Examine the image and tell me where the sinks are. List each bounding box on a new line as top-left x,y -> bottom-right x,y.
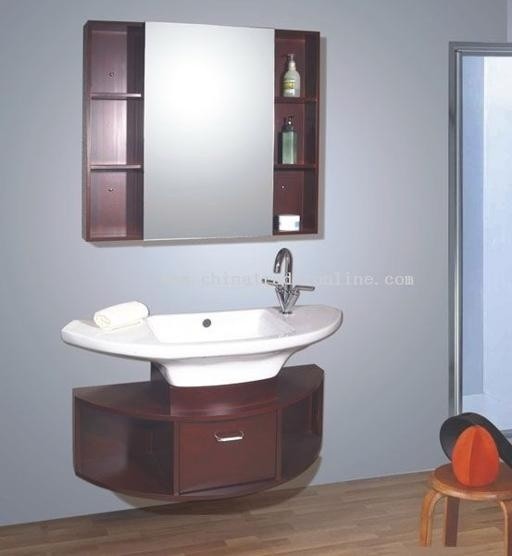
61,304 -> 344,388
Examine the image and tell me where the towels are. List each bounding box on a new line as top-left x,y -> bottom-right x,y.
94,300 -> 149,332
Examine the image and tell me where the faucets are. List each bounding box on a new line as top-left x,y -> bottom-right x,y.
261,248 -> 315,313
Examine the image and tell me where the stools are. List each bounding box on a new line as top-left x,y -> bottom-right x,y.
418,460 -> 511,556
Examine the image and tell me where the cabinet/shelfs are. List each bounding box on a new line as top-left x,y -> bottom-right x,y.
71,364 -> 325,502
273,28 -> 320,236
82,20 -> 144,243
